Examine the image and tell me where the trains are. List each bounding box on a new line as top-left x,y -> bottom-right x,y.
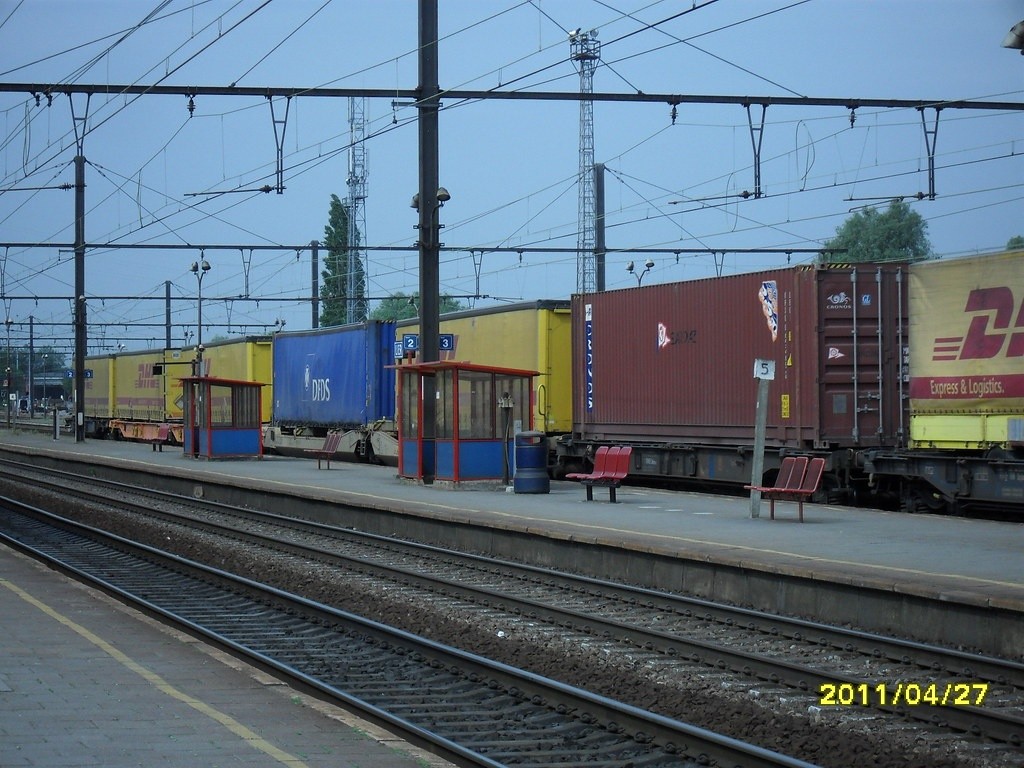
70,234 -> 1023,522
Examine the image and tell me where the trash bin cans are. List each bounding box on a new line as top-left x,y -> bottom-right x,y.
514,431 -> 550,494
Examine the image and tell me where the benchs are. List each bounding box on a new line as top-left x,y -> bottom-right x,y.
302,433 -> 342,469
565,446 -> 632,504
743,457 -> 825,523
150,422 -> 171,452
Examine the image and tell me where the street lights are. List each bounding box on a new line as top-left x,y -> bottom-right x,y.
4,317 -> 15,428
410,187 -> 451,364
190,260 -> 212,377
